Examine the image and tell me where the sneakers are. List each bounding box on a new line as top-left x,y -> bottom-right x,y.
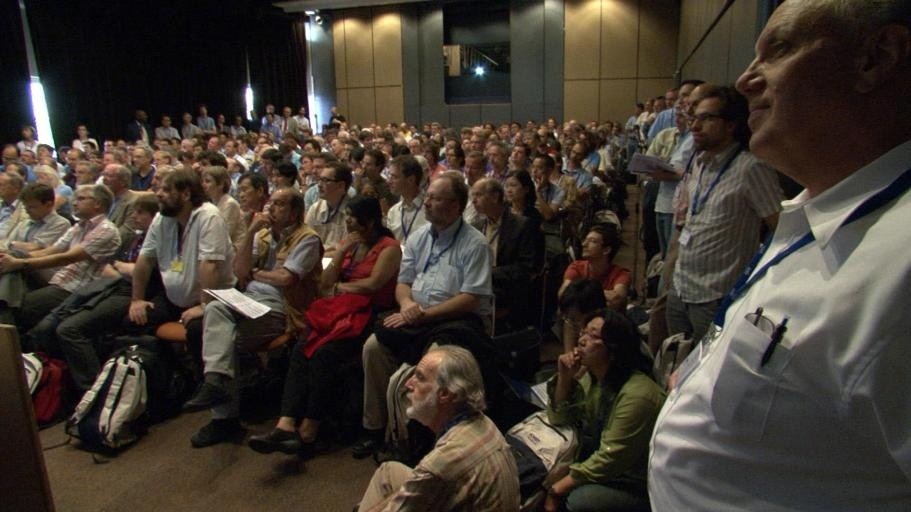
183,381 -> 232,412
191,417 -> 244,447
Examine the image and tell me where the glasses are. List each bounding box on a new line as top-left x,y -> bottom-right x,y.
316,176 -> 336,182
78,196 -> 95,201
579,328 -> 602,339
745,306 -> 774,337
688,111 -> 722,121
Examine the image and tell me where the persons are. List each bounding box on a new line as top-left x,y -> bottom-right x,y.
351,170 -> 494,463
562,142 -> 593,197
556,227 -> 631,352
228,104 -> 321,187
0,185 -> 122,331
237,172 -> 271,238
385,156 -> 429,244
627,79 -> 678,257
305,163 -> 353,259
0,182 -> 70,317
461,150 -> 488,232
0,172 -> 29,247
472,177 -> 535,305
530,153 -> 566,279
201,166 -> 247,255
646,1 -> 911,511
124,168 -> 238,382
545,152 -> 577,212
248,193 -> 402,455
30,194 -> 160,383
100,163 -> 140,227
304,152 -> 355,217
33,164 -> 73,223
323,106 -> 622,156
546,306 -> 667,512
502,168 -> 541,237
0,110 -> 228,165
666,85 -> 787,349
357,344 -> 521,511
73,159 -> 100,187
182,187 -> 325,446
559,280 -> 655,377
351,151 -> 392,207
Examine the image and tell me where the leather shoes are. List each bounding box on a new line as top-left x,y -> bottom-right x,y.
352,437 -> 371,454
247,427 -> 297,453
280,439 -> 316,457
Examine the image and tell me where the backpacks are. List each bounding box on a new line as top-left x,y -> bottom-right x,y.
46,343 -> 157,465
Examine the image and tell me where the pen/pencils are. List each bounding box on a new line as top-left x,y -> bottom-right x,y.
758,317 -> 789,367
